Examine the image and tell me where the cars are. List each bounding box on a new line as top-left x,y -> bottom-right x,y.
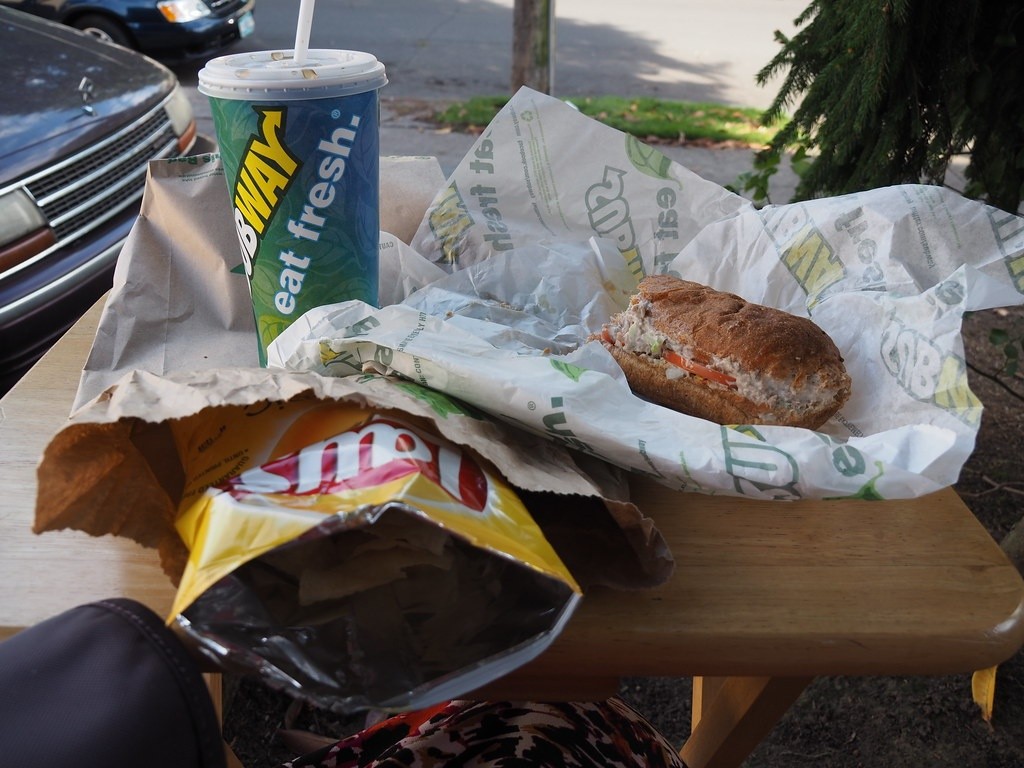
0,4 -> 219,399
6,0 -> 262,68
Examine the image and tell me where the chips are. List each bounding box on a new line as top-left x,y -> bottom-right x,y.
260,521 -> 458,607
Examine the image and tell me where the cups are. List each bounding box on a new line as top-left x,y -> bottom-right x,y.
198,49 -> 389,370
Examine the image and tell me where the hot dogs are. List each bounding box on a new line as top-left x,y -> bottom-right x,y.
596,281 -> 851,428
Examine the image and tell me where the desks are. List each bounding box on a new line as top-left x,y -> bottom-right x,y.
0,287 -> 1024,768
